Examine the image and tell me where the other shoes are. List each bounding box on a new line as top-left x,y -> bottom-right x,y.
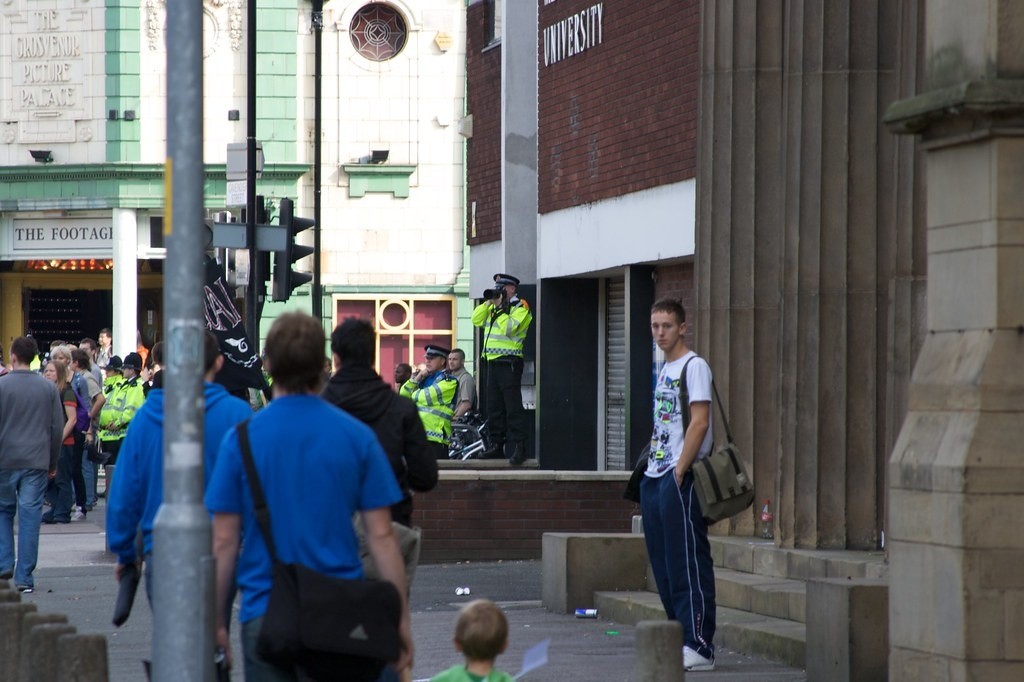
41,508 -> 55,524
682,646 -> 715,671
52,510 -> 72,524
510,447 -> 528,464
478,442 -> 505,459
0,570 -> 13,580
70,511 -> 87,522
17,585 -> 33,593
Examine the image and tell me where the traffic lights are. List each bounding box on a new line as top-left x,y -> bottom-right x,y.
272,196 -> 314,304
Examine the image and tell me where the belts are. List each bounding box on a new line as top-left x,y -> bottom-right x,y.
484,360 -> 523,368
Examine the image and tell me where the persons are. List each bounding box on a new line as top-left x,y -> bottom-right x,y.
398,599 -> 514,682
394,344 -> 475,459
471,273 -> 533,465
0,337 -> 64,591
105,310 -> 439,682
0,328 -> 167,524
639,298 -> 715,671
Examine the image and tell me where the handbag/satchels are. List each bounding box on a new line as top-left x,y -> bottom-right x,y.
255,562 -> 402,682
691,444 -> 756,527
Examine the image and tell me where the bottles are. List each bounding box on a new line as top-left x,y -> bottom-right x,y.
761,499 -> 773,538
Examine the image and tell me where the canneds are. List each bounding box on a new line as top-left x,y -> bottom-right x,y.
575,608 -> 598,618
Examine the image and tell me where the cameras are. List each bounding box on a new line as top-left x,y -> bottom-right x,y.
484,286 -> 507,301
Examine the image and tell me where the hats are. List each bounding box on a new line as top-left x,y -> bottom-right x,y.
100,356 -> 123,370
424,345 -> 451,360
494,274 -> 520,287
86,445 -> 112,465
121,352 -> 142,371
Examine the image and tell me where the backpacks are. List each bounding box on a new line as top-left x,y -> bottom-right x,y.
60,386 -> 90,432
